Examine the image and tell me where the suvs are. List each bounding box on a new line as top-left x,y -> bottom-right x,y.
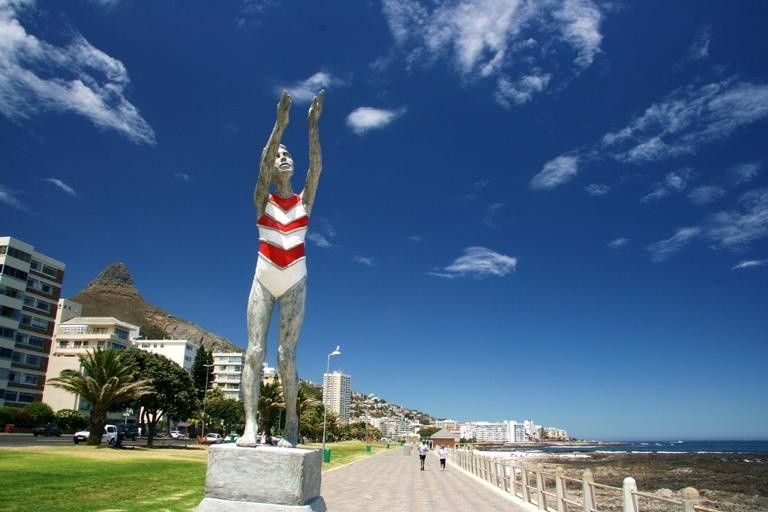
74,424 -> 118,445
117,425 -> 138,441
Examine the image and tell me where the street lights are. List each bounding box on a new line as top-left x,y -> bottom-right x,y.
204,365 -> 213,417
322,345 -> 342,459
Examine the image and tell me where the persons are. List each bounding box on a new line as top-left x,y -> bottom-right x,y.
235,88 -> 327,447
417,439 -> 429,470
437,444 -> 448,471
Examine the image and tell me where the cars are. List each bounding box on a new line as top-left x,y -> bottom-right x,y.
172,431 -> 186,439
224,433 -> 240,442
33,423 -> 62,437
202,433 -> 222,444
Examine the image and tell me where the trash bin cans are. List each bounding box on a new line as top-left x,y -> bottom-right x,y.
324,448 -> 330,463
367,445 -> 372,452
387,444 -> 389,448
